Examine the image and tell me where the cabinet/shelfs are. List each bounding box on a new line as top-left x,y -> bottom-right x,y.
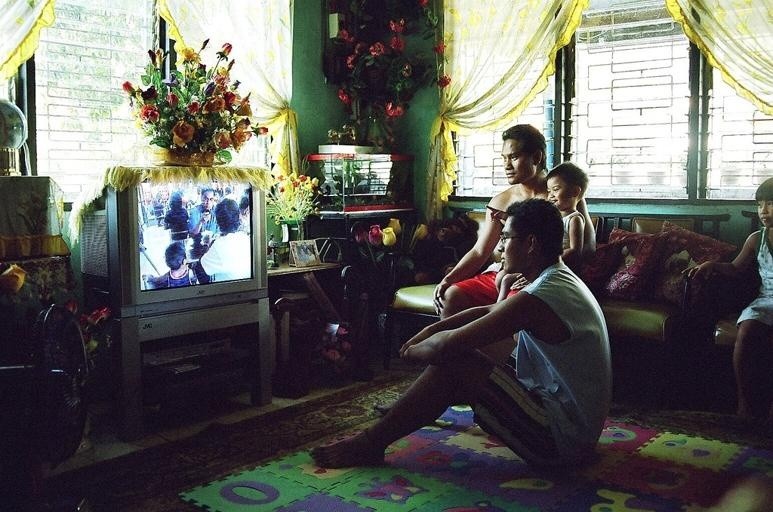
307,145 -> 413,243
114,297 -> 274,443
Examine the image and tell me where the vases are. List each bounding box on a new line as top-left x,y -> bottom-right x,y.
376,311 -> 393,335
287,226 -> 304,241
164,150 -> 215,167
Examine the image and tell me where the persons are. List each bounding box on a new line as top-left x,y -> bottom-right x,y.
680,177 -> 773,419
308,197 -> 614,470
433,122 -> 597,321
142,184 -> 251,288
485,161 -> 590,303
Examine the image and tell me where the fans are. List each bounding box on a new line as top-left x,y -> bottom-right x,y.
26,305 -> 89,512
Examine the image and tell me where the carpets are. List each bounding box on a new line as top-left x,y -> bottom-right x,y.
0,366 -> 773,512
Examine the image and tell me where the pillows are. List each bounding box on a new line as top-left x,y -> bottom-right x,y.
648,220 -> 736,308
565,243 -> 618,292
603,226 -> 663,300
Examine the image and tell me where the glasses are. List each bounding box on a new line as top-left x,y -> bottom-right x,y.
500,235 -> 521,243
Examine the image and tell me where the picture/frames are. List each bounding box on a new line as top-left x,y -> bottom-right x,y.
289,239 -> 322,268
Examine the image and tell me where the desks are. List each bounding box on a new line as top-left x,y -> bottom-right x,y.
268,261 -> 342,383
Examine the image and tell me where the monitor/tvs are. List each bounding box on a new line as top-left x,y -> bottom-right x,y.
80,166 -> 268,318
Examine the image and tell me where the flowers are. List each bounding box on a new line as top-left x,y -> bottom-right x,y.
317,319 -> 352,387
350,218 -> 450,287
325,0 -> 449,153
267,171 -> 322,225
123,37 -> 268,163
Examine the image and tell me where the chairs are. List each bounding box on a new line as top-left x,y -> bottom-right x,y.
597,213 -> 732,384
710,212 -> 758,402
388,211 -> 601,383
